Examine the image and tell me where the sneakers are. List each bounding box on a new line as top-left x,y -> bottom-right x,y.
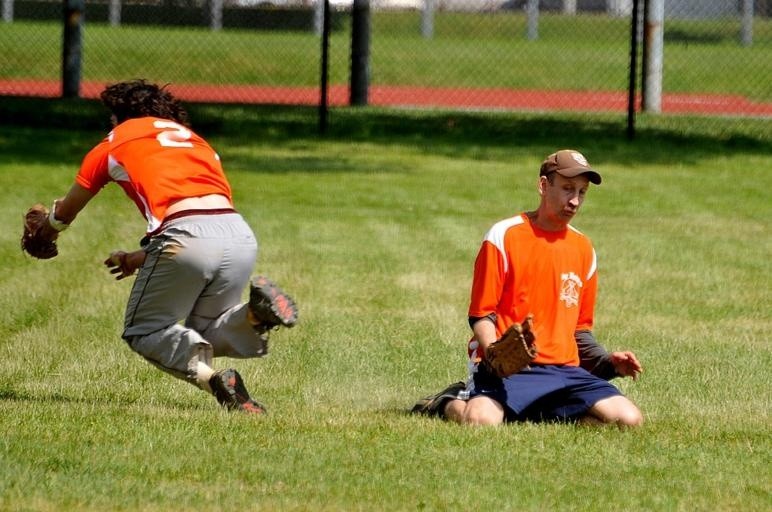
209,368 -> 267,414
249,275 -> 299,328
410,381 -> 467,417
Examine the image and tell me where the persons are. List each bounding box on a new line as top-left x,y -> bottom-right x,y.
408,149 -> 644,430
22,78 -> 299,414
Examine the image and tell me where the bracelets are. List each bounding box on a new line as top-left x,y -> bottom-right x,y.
47,199 -> 70,231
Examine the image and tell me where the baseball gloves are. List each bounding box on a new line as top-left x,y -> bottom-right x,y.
488,313 -> 536,379
21,203 -> 58,259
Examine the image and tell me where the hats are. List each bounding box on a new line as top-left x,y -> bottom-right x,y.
540,150 -> 601,186
